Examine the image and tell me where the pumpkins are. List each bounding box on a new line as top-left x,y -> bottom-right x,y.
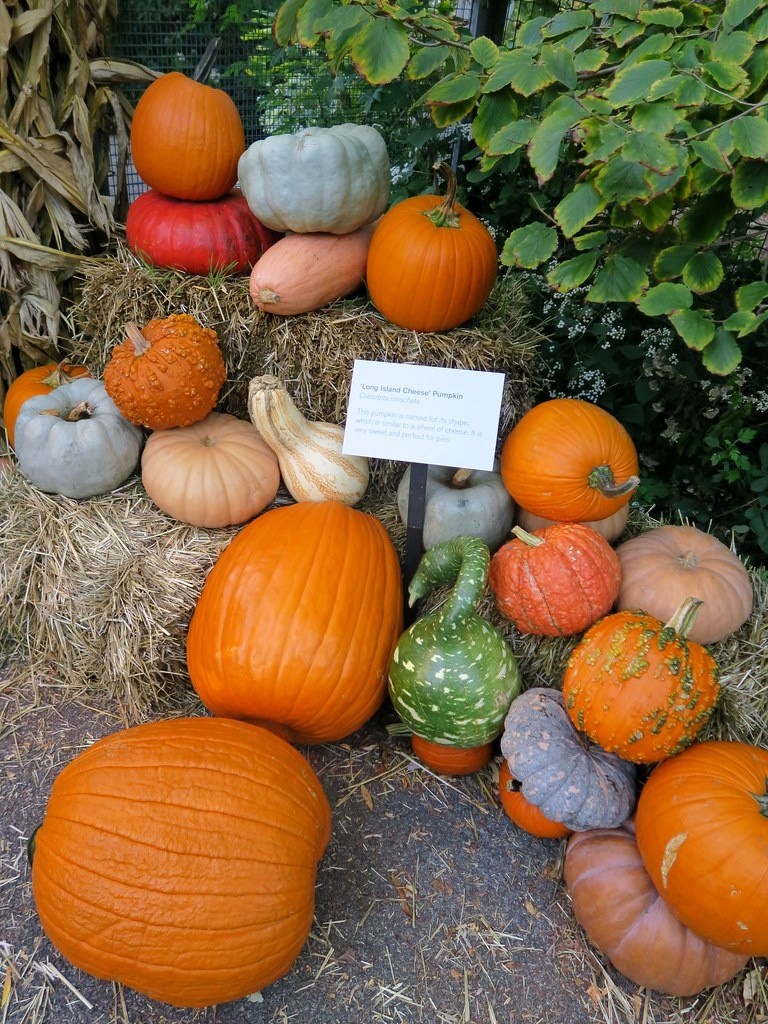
4,35 -> 768,1009
